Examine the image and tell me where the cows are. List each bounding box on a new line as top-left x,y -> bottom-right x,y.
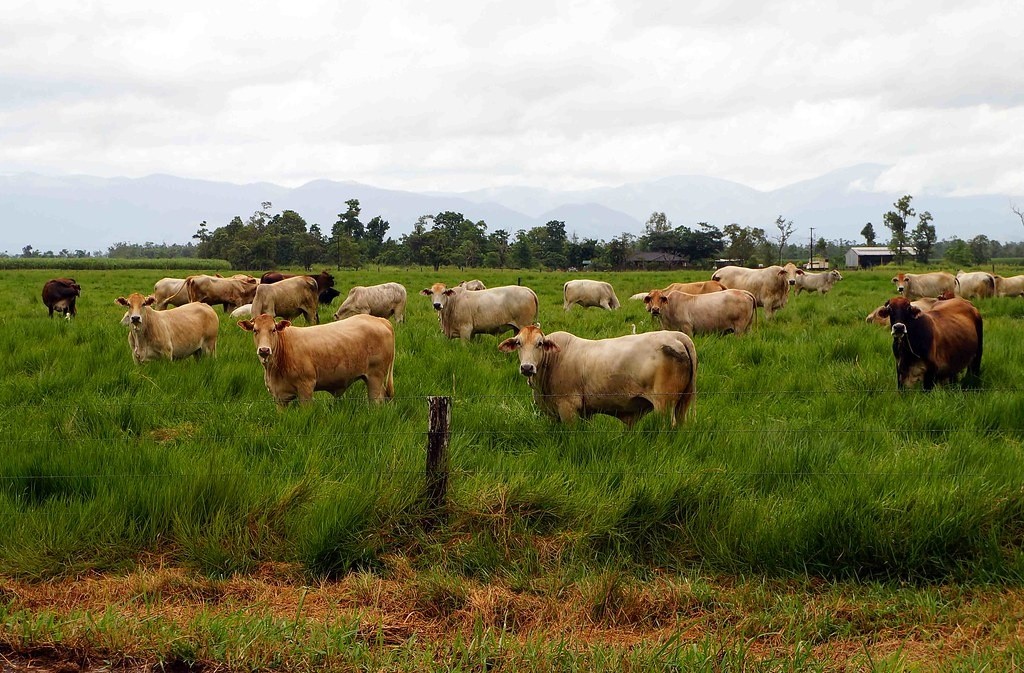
419,283 -> 540,344
497,322 -> 698,432
456,279 -> 486,291
152,270 -> 340,314
956,269 -> 996,301
878,296 -> 985,394
42,278 -> 81,320
113,293 -> 219,366
563,279 -> 621,314
795,269 -> 843,296
333,283 -> 406,324
712,262 -> 806,321
991,274 -> 1024,298
643,281 -> 760,339
237,314 -> 396,413
863,297 -> 939,327
891,271 -> 961,301
